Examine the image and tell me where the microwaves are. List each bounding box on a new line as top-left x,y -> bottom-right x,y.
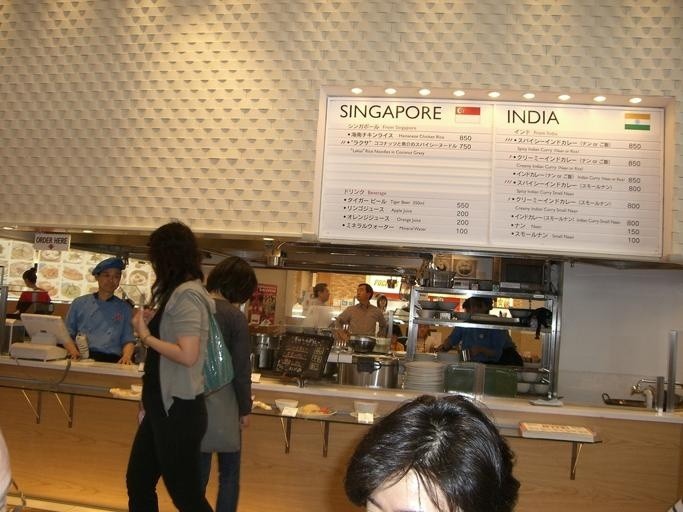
501,259 -> 551,291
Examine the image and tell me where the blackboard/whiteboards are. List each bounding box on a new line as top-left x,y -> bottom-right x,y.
273,332 -> 334,381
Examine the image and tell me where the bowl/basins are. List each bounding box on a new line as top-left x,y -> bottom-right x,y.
439,302 -> 458,310
346,339 -> 376,354
534,384 -> 550,395
516,383 -> 531,393
416,310 -> 435,319
523,373 -> 537,381
516,372 -> 523,382
275,399 -> 298,410
420,300 -> 442,309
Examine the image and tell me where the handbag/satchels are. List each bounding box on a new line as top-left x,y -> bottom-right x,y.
202,315 -> 234,396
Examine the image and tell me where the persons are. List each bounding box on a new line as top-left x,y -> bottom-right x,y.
125,297 -> 157,327
127,222 -> 213,512
199,256 -> 258,509
302,283 -> 330,316
14,268 -> 51,342
377,294 -> 387,313
344,393 -> 519,511
63,257 -> 137,366
335,283 -> 388,341
405,323 -> 435,353
431,296 -> 523,365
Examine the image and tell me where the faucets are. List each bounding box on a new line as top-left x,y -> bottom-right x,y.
630,384 -> 653,408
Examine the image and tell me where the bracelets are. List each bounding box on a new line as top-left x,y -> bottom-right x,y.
141,335 -> 150,349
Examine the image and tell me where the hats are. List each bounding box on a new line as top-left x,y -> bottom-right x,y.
92,257 -> 125,275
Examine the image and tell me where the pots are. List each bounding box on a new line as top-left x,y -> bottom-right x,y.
252,335 -> 276,369
337,355 -> 400,389
429,268 -> 456,288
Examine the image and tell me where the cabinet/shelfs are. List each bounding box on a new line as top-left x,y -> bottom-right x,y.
406,286 -> 557,397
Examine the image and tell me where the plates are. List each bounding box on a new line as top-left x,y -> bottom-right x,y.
403,361 -> 445,392
304,410 -> 339,418
349,411 -> 380,419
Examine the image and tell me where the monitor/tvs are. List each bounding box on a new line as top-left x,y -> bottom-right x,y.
20,313 -> 74,347
